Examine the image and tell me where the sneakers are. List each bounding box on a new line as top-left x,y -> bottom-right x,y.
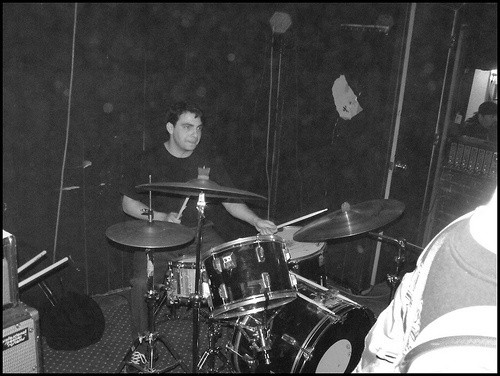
126,343 -> 157,374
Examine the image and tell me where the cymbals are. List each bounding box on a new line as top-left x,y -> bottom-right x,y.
293,198 -> 406,241
106,220 -> 194,248
137,177 -> 268,201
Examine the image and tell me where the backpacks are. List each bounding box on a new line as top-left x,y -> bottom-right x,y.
20,270 -> 104,351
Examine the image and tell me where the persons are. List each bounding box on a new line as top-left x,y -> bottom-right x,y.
351,188 -> 497,373
122,102 -> 278,373
321,71 -> 413,283
447,100 -> 497,163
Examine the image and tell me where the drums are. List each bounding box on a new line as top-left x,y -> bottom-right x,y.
257,226 -> 327,288
200,235 -> 298,320
229,282 -> 376,373
168,256 -> 212,308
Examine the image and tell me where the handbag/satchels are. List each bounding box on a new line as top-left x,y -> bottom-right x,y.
352,267 -> 408,373
